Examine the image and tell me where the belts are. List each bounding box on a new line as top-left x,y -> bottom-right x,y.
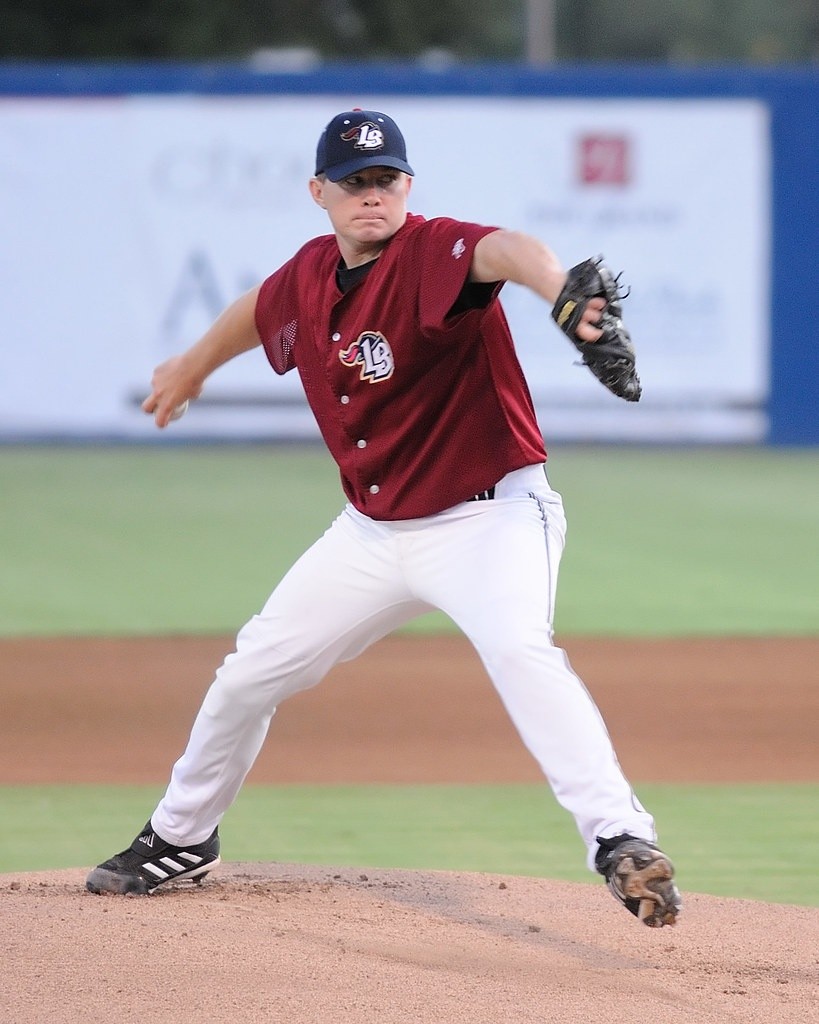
467,486 -> 494,501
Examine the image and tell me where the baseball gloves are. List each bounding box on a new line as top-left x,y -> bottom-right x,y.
549,255 -> 643,403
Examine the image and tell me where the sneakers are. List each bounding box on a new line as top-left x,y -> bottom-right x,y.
88,821 -> 221,894
594,835 -> 682,928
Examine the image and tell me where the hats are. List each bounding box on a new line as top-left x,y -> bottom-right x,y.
316,109 -> 415,182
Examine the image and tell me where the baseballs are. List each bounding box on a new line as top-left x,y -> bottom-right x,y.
153,398 -> 190,423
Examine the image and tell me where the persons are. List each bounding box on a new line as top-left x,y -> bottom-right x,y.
84,106 -> 680,929
